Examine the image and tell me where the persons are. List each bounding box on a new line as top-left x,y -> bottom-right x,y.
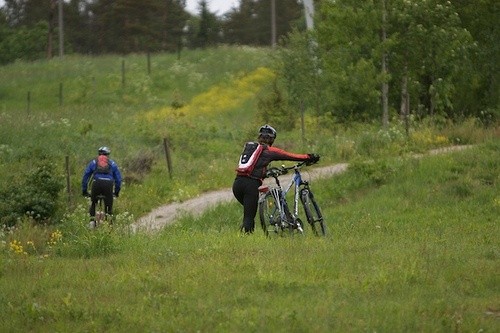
81,146 -> 122,231
232,125 -> 320,237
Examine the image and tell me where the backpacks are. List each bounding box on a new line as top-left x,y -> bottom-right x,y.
96,155 -> 114,175
238,141 -> 268,178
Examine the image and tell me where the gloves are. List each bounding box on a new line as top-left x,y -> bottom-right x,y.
114,191 -> 119,197
306,153 -> 320,163
83,192 -> 90,197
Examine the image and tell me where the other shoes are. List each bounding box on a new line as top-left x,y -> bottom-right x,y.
90,221 -> 95,229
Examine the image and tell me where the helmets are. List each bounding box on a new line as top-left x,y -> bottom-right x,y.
98,146 -> 111,154
258,124 -> 276,138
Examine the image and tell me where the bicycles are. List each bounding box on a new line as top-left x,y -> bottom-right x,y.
258,155 -> 324,239
84,194 -> 116,227
